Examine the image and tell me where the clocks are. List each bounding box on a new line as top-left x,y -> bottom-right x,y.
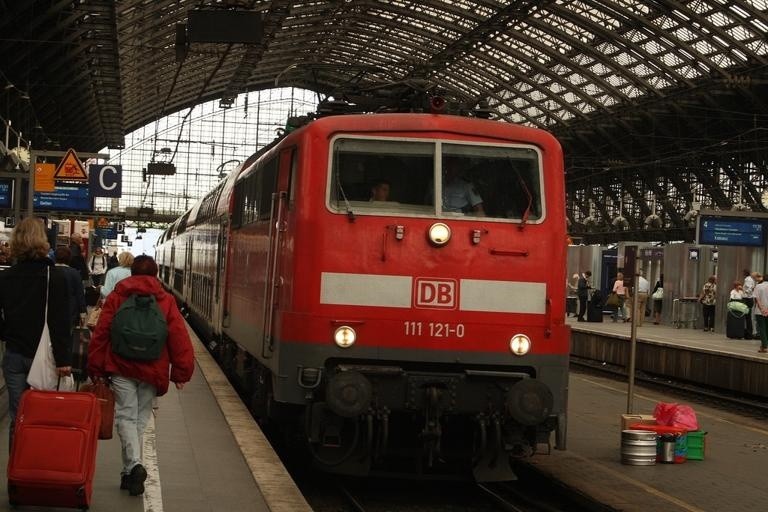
10,147 -> 30,169
759,189 -> 768,212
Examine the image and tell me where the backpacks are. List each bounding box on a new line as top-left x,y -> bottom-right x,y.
108,293 -> 169,362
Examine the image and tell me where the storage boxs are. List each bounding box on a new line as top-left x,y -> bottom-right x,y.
658,427 -> 708,461
622,414 -> 656,430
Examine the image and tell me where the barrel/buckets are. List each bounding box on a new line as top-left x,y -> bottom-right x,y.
622,430 -> 657,465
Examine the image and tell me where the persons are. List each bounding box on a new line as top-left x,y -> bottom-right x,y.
756,274 -> 767,352
89,252 -> 196,495
730,266 -> 766,340
0,218 -> 74,460
697,276 -> 717,332
53,227 -> 136,351
366,179 -> 393,202
424,161 -> 484,220
566,266 -> 666,327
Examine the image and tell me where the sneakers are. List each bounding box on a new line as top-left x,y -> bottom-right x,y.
577,316 -> 715,332
120,464 -> 148,496
758,347 -> 768,353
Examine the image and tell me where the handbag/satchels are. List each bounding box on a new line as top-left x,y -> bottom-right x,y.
78,376 -> 115,440
652,287 -> 664,300
85,305 -> 103,333
590,290 -> 603,306
727,301 -> 750,319
25,322 -> 76,393
605,292 -> 620,307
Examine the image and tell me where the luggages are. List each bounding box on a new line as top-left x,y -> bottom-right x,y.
587,300 -> 603,323
71,326 -> 93,384
6,368 -> 102,512
565,296 -> 578,313
726,310 -> 745,340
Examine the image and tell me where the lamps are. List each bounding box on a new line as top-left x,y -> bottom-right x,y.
567,174 -> 748,229
1,119 -> 31,173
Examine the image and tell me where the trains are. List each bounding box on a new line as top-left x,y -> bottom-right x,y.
152,85 -> 569,486
